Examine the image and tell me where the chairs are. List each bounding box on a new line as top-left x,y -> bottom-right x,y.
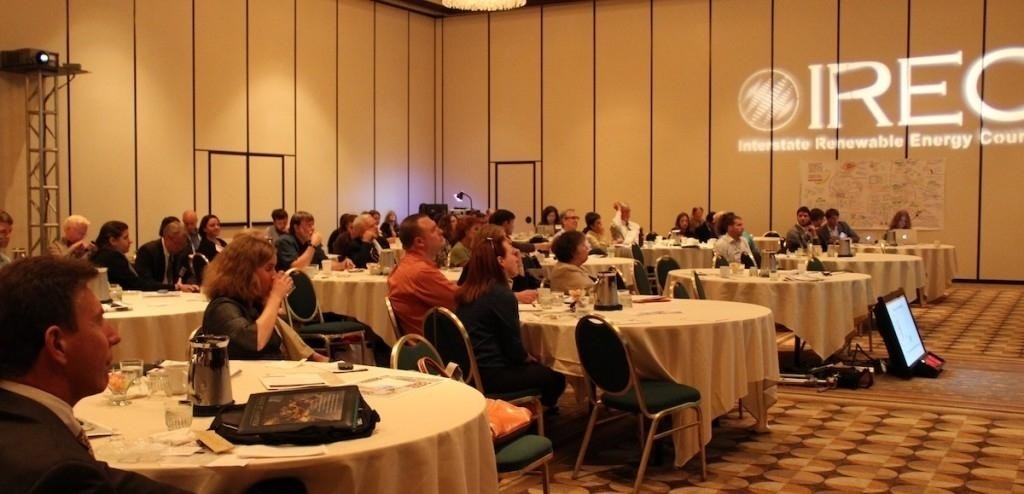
283,266 -> 368,366
193,252 -> 211,285
655,256 -> 682,294
390,332 -> 554,494
670,283 -> 690,299
572,315 -> 707,494
633,258 -> 649,294
424,305 -> 546,437
384,298 -> 404,339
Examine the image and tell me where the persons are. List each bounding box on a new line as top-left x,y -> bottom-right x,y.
551,231 -> 598,292
786,206 -> 821,253
159,216 -> 181,237
438,214 -> 465,246
609,200 -> 640,246
369,210 -> 380,225
553,209 -> 579,238
456,235 -> 567,414
196,215 -> 227,261
447,218 -> 487,267
45,215 -> 95,256
489,209 -> 553,253
182,210 -> 202,250
343,214 -> 391,268
201,233 -> 295,360
277,210 -> 356,274
385,214 -> 463,344
380,211 -> 400,238
688,208 -> 705,234
671,212 -> 693,238
0,210 -> 14,262
694,212 -> 719,243
884,210 -> 911,240
264,208 -> 289,238
0,253 -> 166,493
93,221 -> 138,290
333,215 -> 358,254
536,206 -> 560,236
712,216 -> 758,268
810,208 -> 829,245
582,212 -> 609,251
135,222 -> 200,291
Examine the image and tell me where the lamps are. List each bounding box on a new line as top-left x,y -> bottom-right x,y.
452,191 -> 479,214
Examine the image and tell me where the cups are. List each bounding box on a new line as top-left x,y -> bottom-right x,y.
119,359 -> 144,385
322,259 -> 331,274
148,371 -> 170,401
750,267 -> 757,277
276,270 -> 295,297
165,400 -> 193,433
620,293 -> 631,308
720,265 -> 730,278
163,363 -> 189,395
104,368 -> 136,405
536,287 -> 551,305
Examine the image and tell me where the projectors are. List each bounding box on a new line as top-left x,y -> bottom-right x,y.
0,47 -> 60,74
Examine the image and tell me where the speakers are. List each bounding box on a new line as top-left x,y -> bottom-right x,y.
418,203 -> 448,221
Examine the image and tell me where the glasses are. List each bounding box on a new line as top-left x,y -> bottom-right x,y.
486,236 -> 495,249
564,216 -> 579,220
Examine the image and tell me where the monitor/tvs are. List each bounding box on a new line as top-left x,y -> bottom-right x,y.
873,287 -> 927,380
